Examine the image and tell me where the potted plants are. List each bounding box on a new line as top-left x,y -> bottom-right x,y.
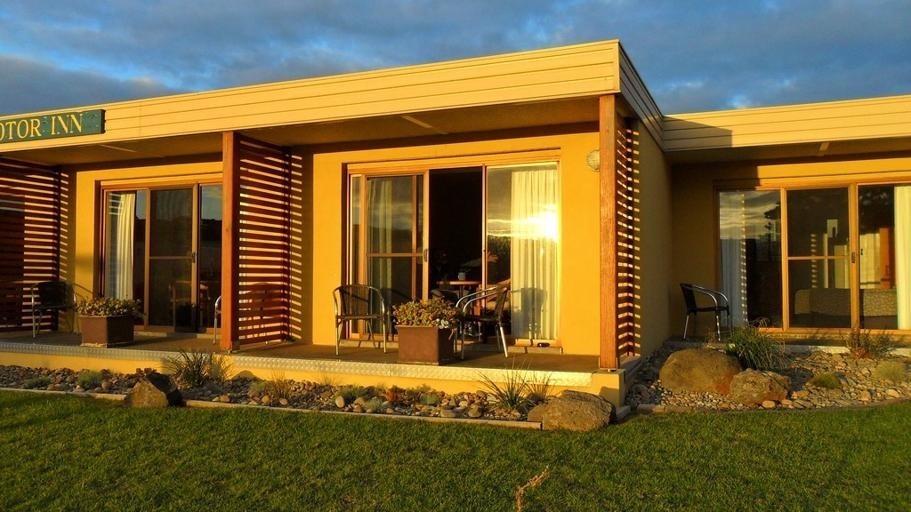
390,299 -> 461,365
77,296 -> 142,348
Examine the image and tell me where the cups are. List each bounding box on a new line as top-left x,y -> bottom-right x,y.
458,271 -> 465,280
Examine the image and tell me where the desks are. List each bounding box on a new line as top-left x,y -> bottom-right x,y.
436,281 -> 479,298
174,279 -> 220,330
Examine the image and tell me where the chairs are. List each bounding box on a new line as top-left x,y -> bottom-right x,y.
213,284 -> 270,344
679,283 -> 733,342
332,284 -> 386,356
453,283 -> 510,361
31,282 -> 77,339
